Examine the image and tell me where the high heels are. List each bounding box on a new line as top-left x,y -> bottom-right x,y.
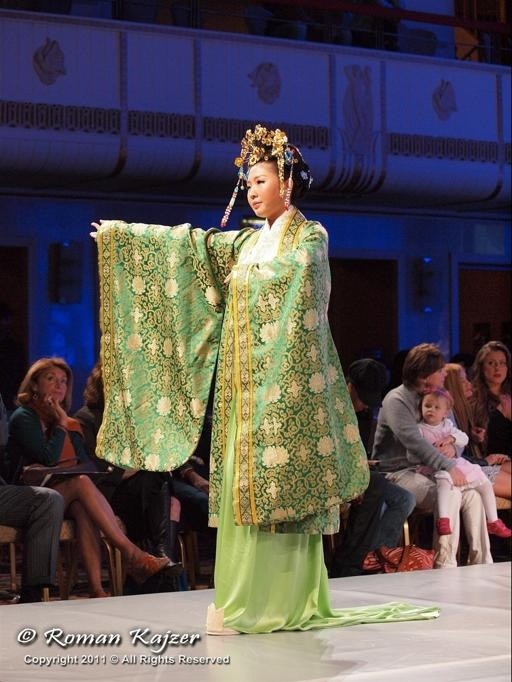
131,555 -> 169,584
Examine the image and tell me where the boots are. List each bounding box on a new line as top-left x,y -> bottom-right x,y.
153,482 -> 184,577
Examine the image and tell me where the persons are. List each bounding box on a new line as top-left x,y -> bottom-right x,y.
88,125 -> 331,634
343,341 -> 512,572
0,357 -> 209,603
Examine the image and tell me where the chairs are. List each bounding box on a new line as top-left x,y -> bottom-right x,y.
1,464 -> 512,601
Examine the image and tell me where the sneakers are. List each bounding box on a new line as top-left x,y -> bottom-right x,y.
487,519 -> 512,537
435,518 -> 451,535
207,602 -> 241,635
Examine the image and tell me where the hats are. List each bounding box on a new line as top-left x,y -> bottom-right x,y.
347,358 -> 391,408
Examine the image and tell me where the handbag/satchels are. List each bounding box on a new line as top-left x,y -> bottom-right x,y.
19,458 -> 106,487
